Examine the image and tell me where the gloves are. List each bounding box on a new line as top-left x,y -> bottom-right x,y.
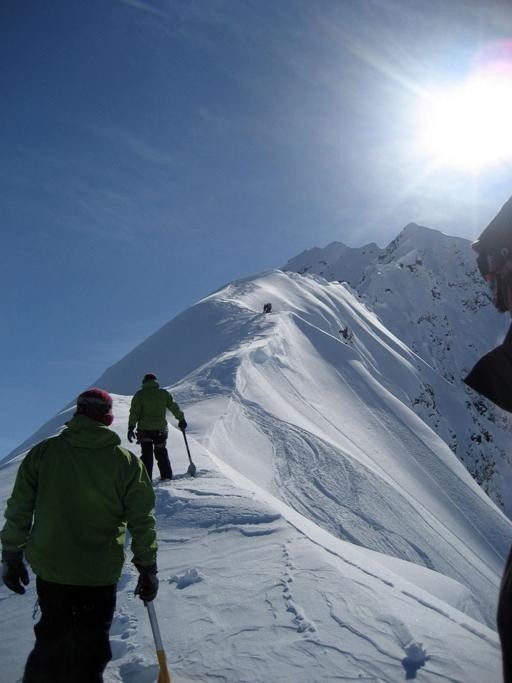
133,565 -> 160,603
177,420 -> 188,430
127,429 -> 136,443
0,550 -> 29,595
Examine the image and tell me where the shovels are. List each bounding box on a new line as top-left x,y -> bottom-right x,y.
183,429 -> 196,476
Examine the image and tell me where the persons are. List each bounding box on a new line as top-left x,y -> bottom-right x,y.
127,373 -> 188,482
0,387 -> 160,683
460,196 -> 512,683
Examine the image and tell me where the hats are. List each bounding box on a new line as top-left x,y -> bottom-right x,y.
74,388 -> 113,426
144,373 -> 157,380
